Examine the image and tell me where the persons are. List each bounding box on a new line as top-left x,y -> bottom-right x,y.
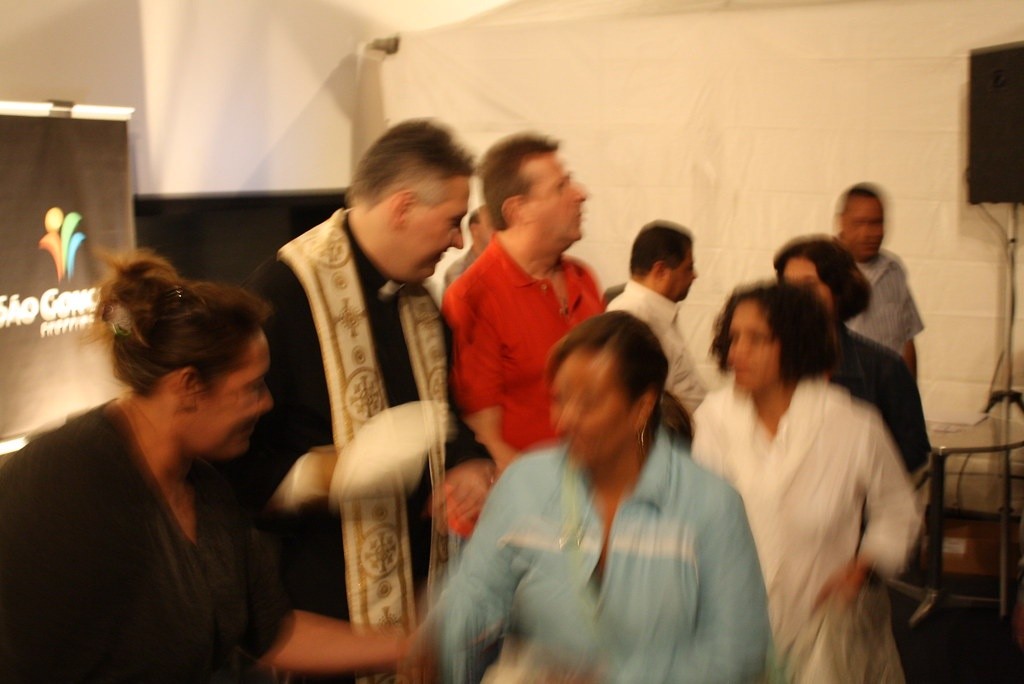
228,115 -> 495,683
440,133 -> 603,485
691,282 -> 922,684
607,221 -> 708,413
443,208 -> 493,283
400,311 -> 768,684
836,184 -> 923,384
772,236 -> 932,560
1,255 -> 407,684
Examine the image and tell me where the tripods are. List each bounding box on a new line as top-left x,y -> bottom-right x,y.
909,202 -> 1024,621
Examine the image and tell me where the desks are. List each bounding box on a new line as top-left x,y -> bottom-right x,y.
892,417 -> 1024,628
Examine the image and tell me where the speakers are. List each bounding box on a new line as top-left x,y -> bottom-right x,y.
966,42 -> 1024,205
